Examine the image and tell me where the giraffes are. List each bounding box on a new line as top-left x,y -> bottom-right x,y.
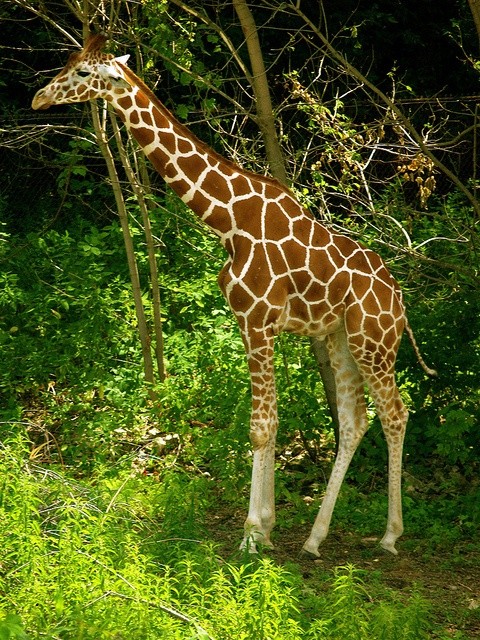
28,19 -> 439,562
